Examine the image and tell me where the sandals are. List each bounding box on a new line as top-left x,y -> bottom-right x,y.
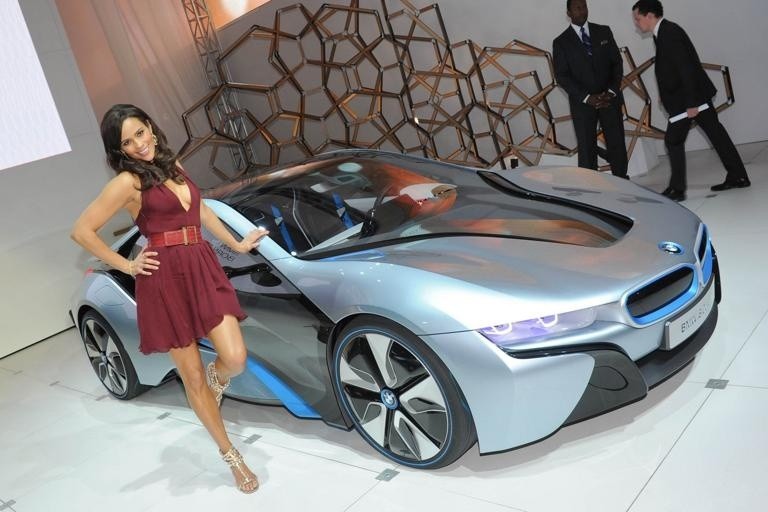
221,447 -> 259,494
206,361 -> 230,406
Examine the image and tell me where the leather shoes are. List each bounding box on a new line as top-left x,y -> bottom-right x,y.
661,188 -> 686,202
710,178 -> 751,191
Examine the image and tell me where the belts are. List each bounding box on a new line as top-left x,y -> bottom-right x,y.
146,226 -> 202,248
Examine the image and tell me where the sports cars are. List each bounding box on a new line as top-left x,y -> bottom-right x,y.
63,146 -> 723,472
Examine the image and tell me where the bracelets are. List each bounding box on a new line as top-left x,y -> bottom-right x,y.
129,260 -> 136,281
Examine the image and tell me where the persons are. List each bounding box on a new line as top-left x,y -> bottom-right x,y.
631,0 -> 751,203
551,0 -> 629,181
69,102 -> 271,493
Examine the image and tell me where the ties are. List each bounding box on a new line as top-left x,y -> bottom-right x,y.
578,27 -> 591,57
